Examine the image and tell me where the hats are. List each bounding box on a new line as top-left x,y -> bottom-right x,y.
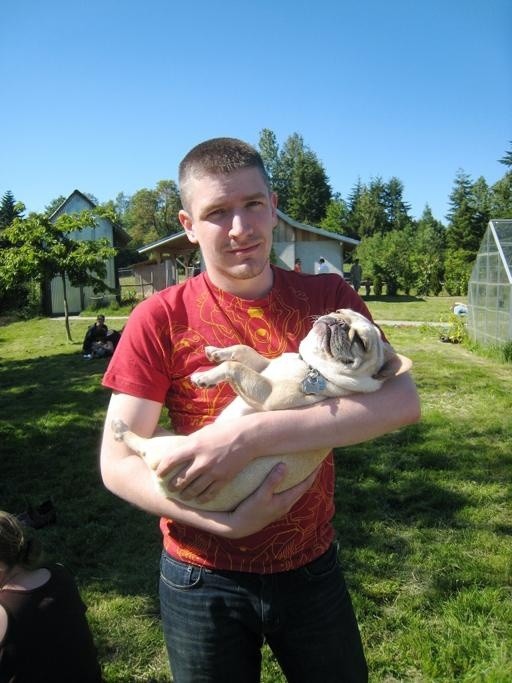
97,315 -> 105,321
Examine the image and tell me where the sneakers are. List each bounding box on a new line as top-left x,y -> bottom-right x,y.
84,354 -> 94,360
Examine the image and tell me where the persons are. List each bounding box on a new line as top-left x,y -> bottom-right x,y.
97,136 -> 425,682
294,257 -> 302,273
0,510 -> 102,683
318,257 -> 329,274
81,313 -> 121,358
349,257 -> 363,293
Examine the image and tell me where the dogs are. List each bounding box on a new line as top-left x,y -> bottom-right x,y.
108,306 -> 413,515
